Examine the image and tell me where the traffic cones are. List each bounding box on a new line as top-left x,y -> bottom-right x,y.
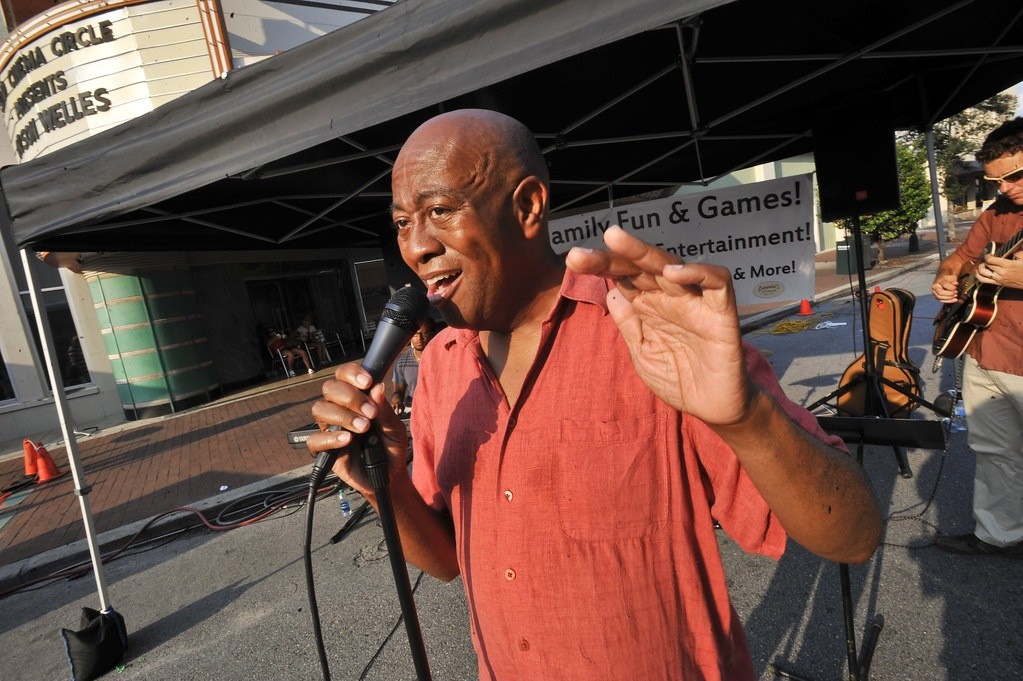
33,440 -> 68,485
22,438 -> 39,476
796,297 -> 815,317
873,286 -> 883,309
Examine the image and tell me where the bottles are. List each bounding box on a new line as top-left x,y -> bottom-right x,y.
941,389 -> 967,434
338,489 -> 352,516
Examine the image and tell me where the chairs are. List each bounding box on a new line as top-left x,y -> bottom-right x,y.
268,326 -> 348,379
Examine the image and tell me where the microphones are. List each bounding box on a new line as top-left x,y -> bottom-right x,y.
307,285 -> 430,488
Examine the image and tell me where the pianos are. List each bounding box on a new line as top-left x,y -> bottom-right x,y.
283,398 -> 421,487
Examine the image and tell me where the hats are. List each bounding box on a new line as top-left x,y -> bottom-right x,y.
304,315 -> 311,322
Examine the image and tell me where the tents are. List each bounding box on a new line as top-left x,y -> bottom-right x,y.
0,0 -> 1023,618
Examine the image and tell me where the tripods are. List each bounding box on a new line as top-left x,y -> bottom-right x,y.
807,218 -> 954,480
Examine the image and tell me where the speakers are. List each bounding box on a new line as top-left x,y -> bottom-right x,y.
810,90 -> 901,224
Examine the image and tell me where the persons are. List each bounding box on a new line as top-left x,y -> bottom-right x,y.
307,109 -> 884,681
267,327 -> 315,377
931,117 -> 1023,554
297,319 -> 331,367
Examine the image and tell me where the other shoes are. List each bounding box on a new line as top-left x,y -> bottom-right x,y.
319,360 -> 330,367
935,533 -> 1023,560
308,368 -> 315,375
289,370 -> 296,377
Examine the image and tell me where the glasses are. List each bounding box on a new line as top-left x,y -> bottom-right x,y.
413,331 -> 432,337
984,166 -> 1023,188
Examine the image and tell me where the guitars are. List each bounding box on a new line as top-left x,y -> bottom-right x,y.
930,227 -> 1023,361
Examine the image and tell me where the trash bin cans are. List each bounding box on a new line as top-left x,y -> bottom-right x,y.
844,234 -> 874,270
